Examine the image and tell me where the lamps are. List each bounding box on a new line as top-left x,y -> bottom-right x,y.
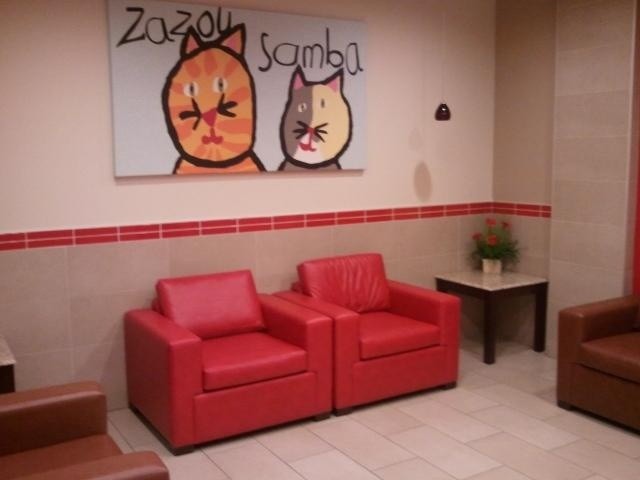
434,1 -> 451,121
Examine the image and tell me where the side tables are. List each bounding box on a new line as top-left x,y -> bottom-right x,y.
433,269 -> 550,365
0,338 -> 15,393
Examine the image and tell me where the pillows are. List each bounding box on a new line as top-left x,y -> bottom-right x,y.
155,270 -> 266,339
296,253 -> 391,313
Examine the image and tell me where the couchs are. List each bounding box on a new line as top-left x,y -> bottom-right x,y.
270,279 -> 461,418
557,293 -> 640,435
0,379 -> 171,480
123,293 -> 334,456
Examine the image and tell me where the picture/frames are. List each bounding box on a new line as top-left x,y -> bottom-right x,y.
107,0 -> 372,180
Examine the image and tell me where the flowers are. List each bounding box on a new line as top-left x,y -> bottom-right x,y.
469,217 -> 524,268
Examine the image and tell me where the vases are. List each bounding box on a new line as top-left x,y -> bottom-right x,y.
482,259 -> 502,274
